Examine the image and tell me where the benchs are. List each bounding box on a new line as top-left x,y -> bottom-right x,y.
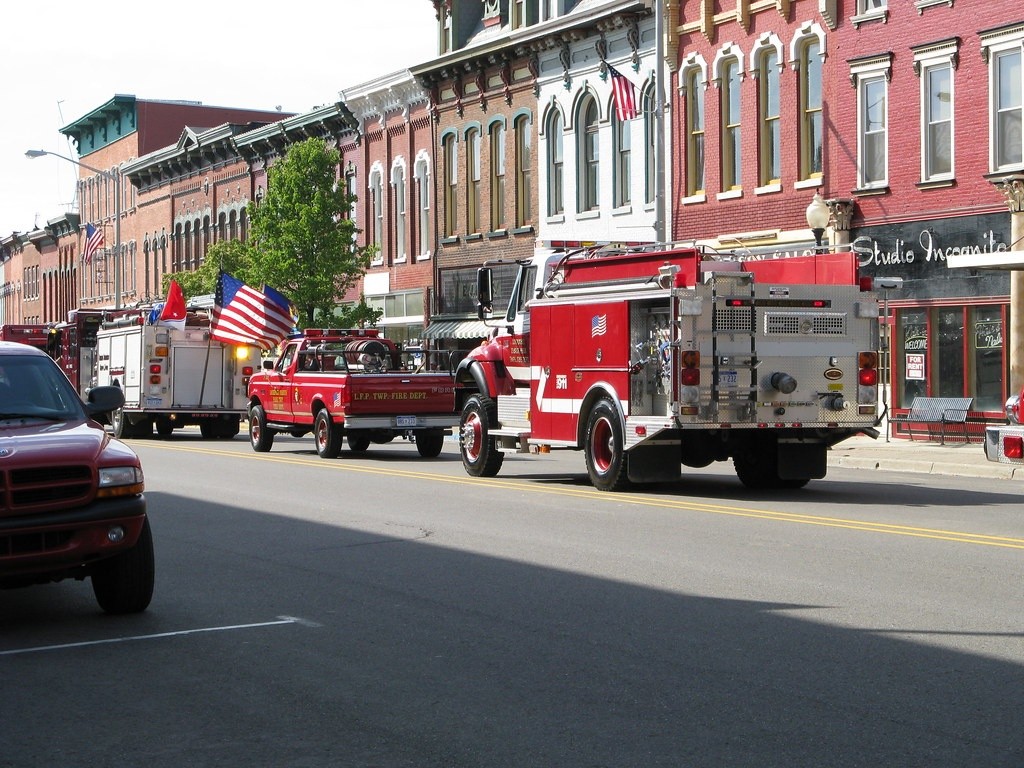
885,397 -> 975,446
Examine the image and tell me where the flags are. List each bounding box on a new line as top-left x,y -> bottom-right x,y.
210,272 -> 297,350
606,65 -> 636,122
83,224 -> 105,263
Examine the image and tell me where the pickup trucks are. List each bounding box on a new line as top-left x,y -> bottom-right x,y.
247,328 -> 465,458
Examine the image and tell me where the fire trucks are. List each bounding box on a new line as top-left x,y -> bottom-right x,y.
453,240 -> 887,499
0,308 -> 262,439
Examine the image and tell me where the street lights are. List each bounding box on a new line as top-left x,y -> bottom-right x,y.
25,150 -> 121,309
805,187 -> 833,451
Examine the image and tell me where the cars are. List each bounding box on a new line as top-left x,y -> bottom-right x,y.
0,341 -> 156,617
